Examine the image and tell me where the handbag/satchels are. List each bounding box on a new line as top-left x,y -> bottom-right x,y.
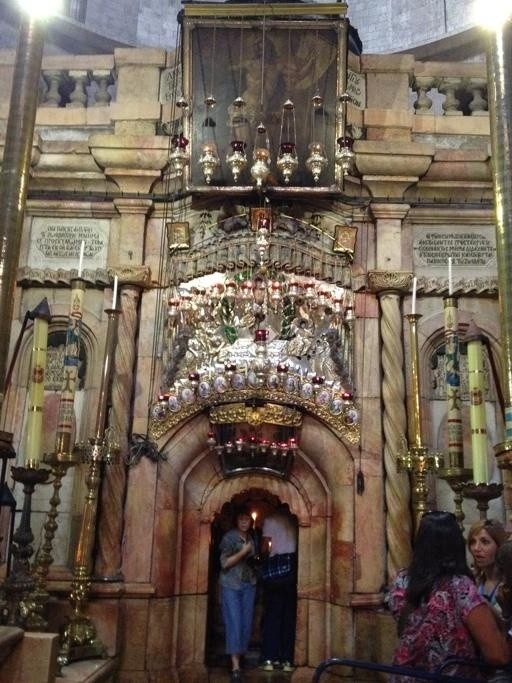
260,554 -> 291,579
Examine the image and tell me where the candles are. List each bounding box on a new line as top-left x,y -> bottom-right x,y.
112,274 -> 118,308
412,276 -> 417,313
77,239 -> 86,277
448,257 -> 453,294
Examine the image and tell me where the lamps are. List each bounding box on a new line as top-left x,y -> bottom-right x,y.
167,13 -> 357,190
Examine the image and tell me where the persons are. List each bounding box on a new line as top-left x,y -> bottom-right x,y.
256,497 -> 295,673
217,510 -> 256,680
492,542 -> 511,635
467,519 -> 506,615
388,509 -> 511,682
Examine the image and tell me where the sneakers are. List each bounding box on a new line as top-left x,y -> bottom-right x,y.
230,659 -> 295,683
